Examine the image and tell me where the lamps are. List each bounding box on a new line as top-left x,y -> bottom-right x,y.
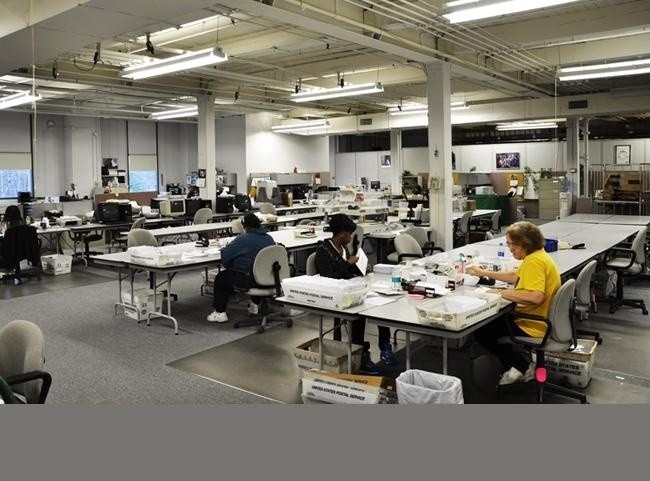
270,108 -> 331,134
390,93 -> 472,115
0,89 -> 41,109
117,15 -> 227,80
146,101 -> 200,122
289,69 -> 385,103
438,0 -> 579,25
557,57 -> 650,82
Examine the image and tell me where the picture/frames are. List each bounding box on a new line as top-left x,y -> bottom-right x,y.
614,144 -> 631,165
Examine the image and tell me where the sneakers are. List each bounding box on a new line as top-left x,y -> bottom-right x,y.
381,344 -> 396,367
248,304 -> 258,314
206,311 -> 229,322
498,365 -> 536,385
360,352 -> 383,374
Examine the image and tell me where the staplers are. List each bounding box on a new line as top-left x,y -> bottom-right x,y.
571,243 -> 586,249
195,242 -> 209,248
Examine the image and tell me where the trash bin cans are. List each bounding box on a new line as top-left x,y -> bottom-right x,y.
532,339 -> 598,388
394,369 -> 466,404
202,269 -> 227,295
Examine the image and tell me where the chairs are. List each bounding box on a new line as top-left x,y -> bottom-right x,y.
82,173 -> 649,403
0,186 -> 81,283
1,317 -> 53,402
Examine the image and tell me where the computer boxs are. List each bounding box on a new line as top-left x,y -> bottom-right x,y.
119,203 -> 132,224
216,197 -> 234,213
186,199 -> 212,217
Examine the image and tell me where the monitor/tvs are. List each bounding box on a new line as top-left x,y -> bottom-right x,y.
96,202 -> 121,224
234,196 -> 251,213
18,191 -> 32,202
160,198 -> 185,216
371,181 -> 380,191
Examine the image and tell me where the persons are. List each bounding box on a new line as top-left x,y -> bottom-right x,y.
1,205 -> 22,240
498,154 -> 517,168
313,213 -> 396,374
470,220 -> 561,385
207,212 -> 276,323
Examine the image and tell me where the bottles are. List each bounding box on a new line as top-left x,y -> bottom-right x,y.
392,266 -> 401,290
496,242 -> 505,263
